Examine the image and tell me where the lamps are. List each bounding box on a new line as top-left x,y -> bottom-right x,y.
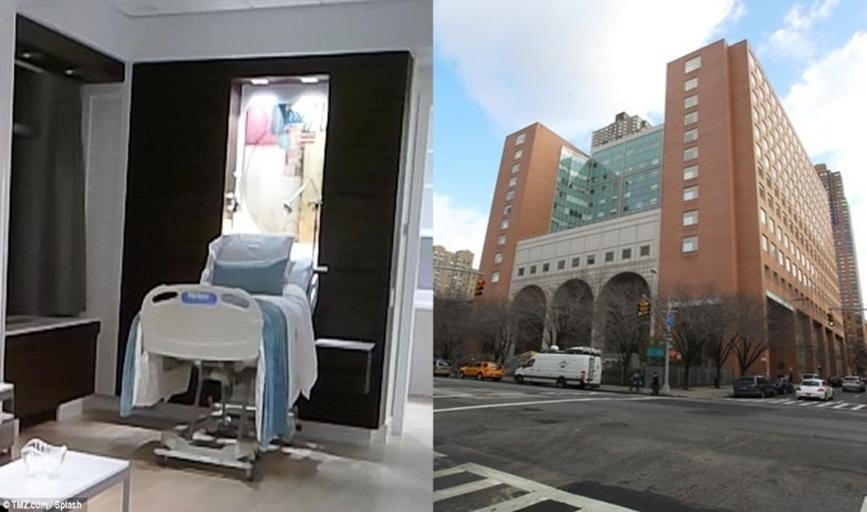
283,176 -> 329,274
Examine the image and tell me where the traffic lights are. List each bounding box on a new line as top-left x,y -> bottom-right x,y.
643,301 -> 651,317
827,313 -> 835,328
635,302 -> 644,317
474,280 -> 485,298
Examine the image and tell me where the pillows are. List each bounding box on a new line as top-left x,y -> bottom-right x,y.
211,233 -> 294,296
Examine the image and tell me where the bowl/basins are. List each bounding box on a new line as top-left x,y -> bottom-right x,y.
21,438 -> 68,477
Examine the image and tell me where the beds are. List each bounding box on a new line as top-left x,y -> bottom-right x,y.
133,231 -> 327,480
0,449 -> 130,510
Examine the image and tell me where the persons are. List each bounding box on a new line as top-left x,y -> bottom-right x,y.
648,370 -> 660,395
626,368 -> 640,392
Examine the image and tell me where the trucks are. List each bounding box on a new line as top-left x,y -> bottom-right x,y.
512,344 -> 604,391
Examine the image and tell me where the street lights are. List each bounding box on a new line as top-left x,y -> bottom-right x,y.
760,356 -> 766,376
649,267 -> 678,397
675,351 -> 684,389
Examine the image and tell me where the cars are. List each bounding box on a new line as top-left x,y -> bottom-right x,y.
459,360 -> 504,383
433,359 -> 450,378
734,370 -> 867,403
